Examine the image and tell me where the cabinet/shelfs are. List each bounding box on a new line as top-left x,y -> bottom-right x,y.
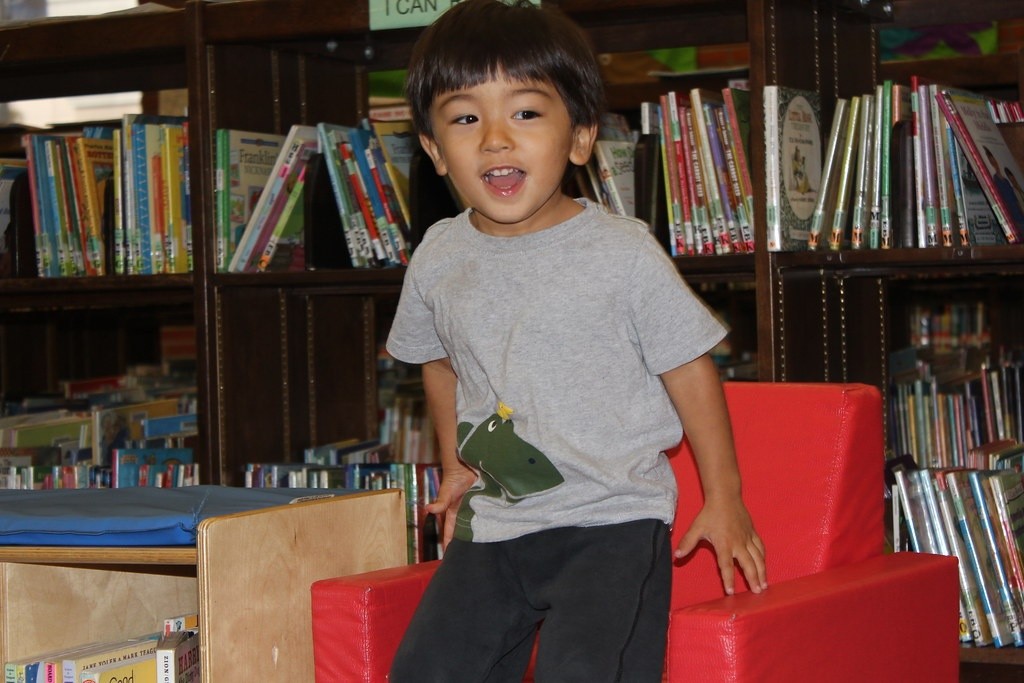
0,0 -> 1024,683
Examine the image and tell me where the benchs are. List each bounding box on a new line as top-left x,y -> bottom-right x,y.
0,484 -> 408,683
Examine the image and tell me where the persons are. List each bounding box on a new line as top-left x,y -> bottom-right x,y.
387,0 -> 768,683
1004,166 -> 1024,210
983,145 -> 1024,228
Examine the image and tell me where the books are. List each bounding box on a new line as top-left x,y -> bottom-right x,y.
569,75 -> 1024,258
216,118 -> 411,272
884,299 -> 1024,648
0,112 -> 193,277
6,613 -> 202,683
0,324 -> 199,489
246,395 -> 445,564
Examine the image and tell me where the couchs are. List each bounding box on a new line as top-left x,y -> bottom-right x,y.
316,379 -> 961,683
